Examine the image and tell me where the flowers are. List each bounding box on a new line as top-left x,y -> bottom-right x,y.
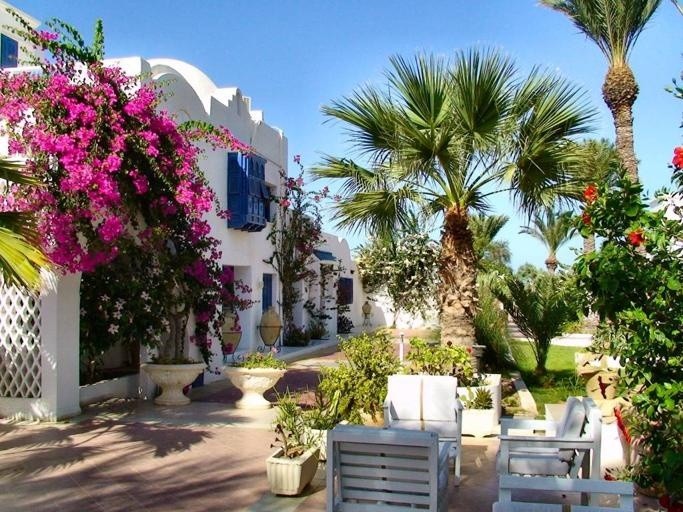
0,1 -> 258,377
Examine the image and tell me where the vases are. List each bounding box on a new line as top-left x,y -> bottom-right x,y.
139,359 -> 211,407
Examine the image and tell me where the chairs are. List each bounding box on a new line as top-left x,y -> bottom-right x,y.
382,374 -> 463,478
497,394 -> 603,512
328,421 -> 450,512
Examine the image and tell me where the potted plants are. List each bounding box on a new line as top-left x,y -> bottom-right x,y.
222,351 -> 287,411
261,333 -> 503,497
